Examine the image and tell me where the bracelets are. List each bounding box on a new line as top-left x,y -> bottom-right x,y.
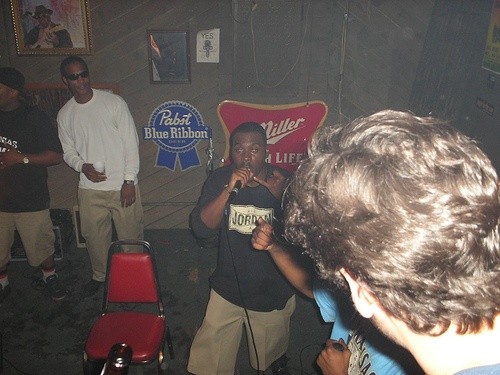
124,180 -> 134,184
224,185 -> 232,193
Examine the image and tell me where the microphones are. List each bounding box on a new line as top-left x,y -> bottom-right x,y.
232,161 -> 252,196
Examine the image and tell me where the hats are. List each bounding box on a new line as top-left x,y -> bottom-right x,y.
0,66 -> 26,92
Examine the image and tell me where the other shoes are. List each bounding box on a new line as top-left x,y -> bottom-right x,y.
271,354 -> 290,375
81,279 -> 103,298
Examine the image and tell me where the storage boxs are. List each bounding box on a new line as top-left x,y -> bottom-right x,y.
74,204 -> 118,248
9,210 -> 72,261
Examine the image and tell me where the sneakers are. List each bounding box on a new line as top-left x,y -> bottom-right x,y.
40,273 -> 68,300
0,282 -> 12,304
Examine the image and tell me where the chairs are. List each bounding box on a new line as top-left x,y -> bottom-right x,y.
83,240 -> 175,375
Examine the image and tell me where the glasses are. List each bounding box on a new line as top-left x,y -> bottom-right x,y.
65,70 -> 89,80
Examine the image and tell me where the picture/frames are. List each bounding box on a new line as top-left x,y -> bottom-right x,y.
10,0 -> 95,56
147,30 -> 191,84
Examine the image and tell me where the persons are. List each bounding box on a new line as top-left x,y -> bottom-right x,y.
250,109 -> 500,375
188,123 -> 303,375
0,69 -> 64,300
23,5 -> 73,50
57,56 -> 141,300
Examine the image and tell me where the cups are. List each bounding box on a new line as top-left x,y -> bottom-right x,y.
93,161 -> 105,175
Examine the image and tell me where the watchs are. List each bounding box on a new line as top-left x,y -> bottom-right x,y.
22,152 -> 30,166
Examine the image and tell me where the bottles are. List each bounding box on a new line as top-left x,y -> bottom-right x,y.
99,343 -> 132,375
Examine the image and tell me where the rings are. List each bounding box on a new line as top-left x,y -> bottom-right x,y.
0,162 -> 3,165
